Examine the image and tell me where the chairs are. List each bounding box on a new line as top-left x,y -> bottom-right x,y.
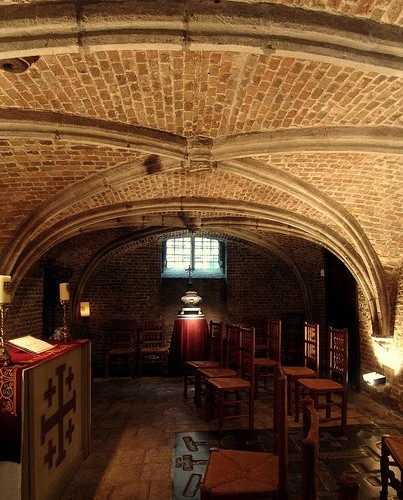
197,366 -> 289,500
197,322 -> 243,422
247,319 -> 282,399
300,395 -> 321,500
104,317 -> 137,379
203,324 -> 256,431
379,432 -> 403,500
183,319 -> 224,405
294,325 -> 350,436
138,318 -> 169,378
281,319 -> 321,421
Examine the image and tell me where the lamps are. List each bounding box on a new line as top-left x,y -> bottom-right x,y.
79,301 -> 91,317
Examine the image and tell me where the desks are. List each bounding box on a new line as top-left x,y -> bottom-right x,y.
174,319 -> 209,377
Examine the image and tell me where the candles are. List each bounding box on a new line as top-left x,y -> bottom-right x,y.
59,282 -> 69,300
0,275 -> 11,303
